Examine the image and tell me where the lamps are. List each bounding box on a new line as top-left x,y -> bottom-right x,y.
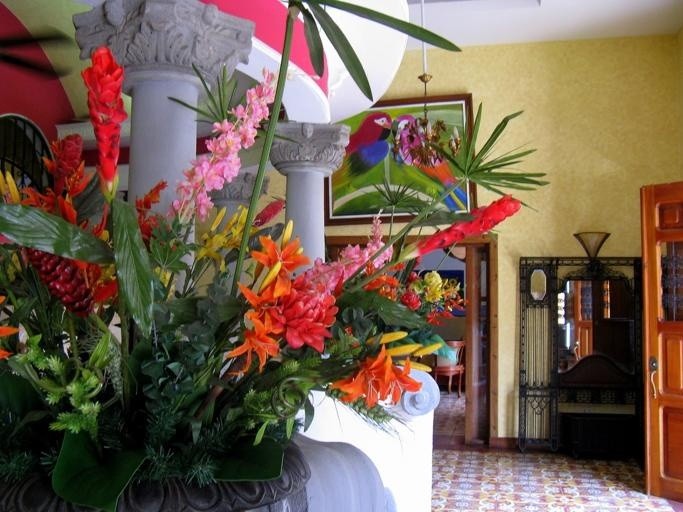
574,232 -> 610,273
392,0 -> 459,170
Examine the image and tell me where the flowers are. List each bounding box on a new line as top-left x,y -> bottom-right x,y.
0,0 -> 549,512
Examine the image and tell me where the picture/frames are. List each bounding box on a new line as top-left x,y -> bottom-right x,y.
325,93 -> 478,227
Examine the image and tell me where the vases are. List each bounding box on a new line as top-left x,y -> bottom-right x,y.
0,440 -> 312,512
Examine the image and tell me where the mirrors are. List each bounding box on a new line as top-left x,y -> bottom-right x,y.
555,256 -> 635,406
525,264 -> 554,308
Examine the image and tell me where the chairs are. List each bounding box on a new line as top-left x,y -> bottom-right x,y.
431,336 -> 466,397
